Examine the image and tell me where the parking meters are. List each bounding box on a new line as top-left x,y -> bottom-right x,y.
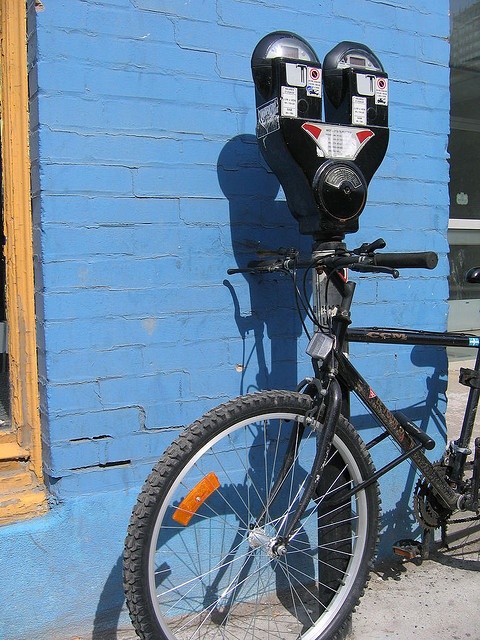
251,31 -> 388,640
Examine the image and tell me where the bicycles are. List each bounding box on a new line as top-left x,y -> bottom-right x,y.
122,238 -> 480,639
